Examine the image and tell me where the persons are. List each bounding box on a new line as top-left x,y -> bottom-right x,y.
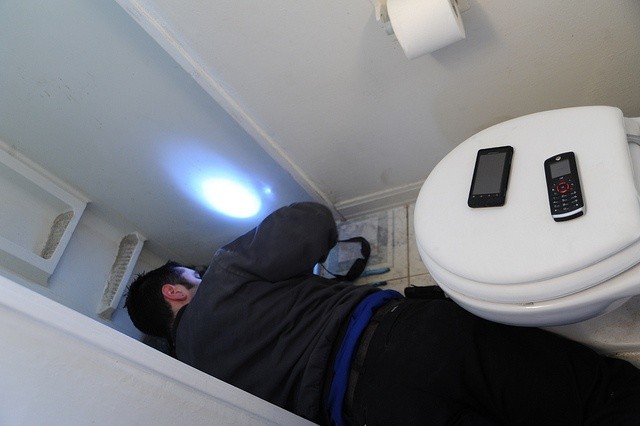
122,201 -> 638,426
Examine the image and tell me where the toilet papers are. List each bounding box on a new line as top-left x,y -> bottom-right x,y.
373,0 -> 468,60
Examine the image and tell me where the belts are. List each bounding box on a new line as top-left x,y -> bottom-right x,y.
341,296 -> 403,426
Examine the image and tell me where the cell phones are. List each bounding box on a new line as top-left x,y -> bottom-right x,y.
543,152 -> 586,222
467,147 -> 514,208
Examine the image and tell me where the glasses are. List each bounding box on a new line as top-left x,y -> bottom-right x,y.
320,236 -> 370,281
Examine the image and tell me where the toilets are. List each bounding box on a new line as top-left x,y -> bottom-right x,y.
413,104 -> 640,350
